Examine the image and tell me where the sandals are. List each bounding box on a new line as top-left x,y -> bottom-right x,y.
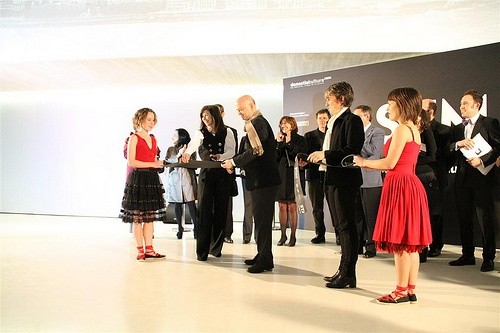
407,283 -> 417,301
137,246 -> 146,261
377,286 -> 409,304
144,245 -> 165,258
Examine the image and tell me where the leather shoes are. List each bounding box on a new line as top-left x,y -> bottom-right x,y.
365,250 -> 375,257
225,237 -> 233,243
336,238 -> 341,244
310,236 -> 326,243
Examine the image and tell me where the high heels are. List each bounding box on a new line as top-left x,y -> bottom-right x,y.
323,269 -> 341,282
277,236 -> 287,245
288,238 -> 296,247
325,274 -> 357,289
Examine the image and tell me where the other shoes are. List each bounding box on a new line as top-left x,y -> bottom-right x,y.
248,262 -> 274,273
243,240 -> 248,244
245,257 -> 256,265
426,248 -> 441,256
481,259 -> 495,272
448,257 -> 475,266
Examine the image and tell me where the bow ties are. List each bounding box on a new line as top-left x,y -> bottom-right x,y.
462,119 -> 473,126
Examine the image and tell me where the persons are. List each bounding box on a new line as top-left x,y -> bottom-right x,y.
298,81 -> 366,289
216,104 -> 238,243
238,132 -> 254,244
164,128 -> 198,239
127,108 -> 166,262
178,105 -> 236,261
220,95 -> 278,274
415,88 -> 500,272
353,105 -> 384,258
276,116 -> 308,245
353,87 -> 430,304
304,108 -> 341,245
